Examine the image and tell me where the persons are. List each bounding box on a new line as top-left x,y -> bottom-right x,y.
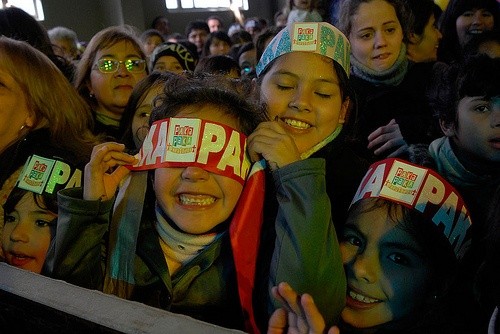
0,142 -> 75,274
151,15 -> 169,36
439,0 -> 500,99
328,0 -> 443,160
70,24 -> 150,156
0,6 -> 57,72
166,33 -> 183,43
426,51 -> 500,333
116,71 -> 185,156
266,145 -> 482,334
236,42 -> 261,78
138,29 -> 165,56
274,10 -> 287,27
244,16 -> 262,35
391,0 -> 495,148
193,55 -> 242,77
467,31 -> 500,62
57,70 -> 348,334
227,24 -> 245,38
202,30 -> 233,56
149,39 -> 199,75
289,0 -> 318,9
255,17 -> 269,33
0,36 -> 117,191
232,31 -> 253,51
46,26 -> 79,61
185,19 -> 211,53
220,21 -> 439,334
205,16 -> 224,33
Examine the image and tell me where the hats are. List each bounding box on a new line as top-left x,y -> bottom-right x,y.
149,40 -> 199,75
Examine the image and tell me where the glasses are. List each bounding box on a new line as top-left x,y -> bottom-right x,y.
91,57 -> 147,73
51,43 -> 80,58
240,63 -> 255,75
